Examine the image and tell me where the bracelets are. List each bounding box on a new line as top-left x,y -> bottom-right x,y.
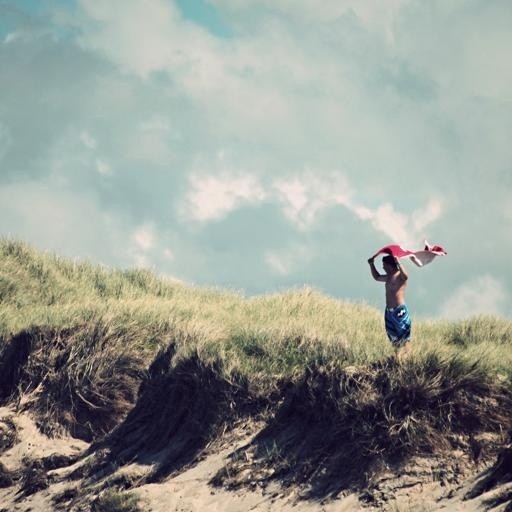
397,262 -> 403,269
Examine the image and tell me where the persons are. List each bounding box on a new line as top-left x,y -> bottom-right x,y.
367,255 -> 414,349
